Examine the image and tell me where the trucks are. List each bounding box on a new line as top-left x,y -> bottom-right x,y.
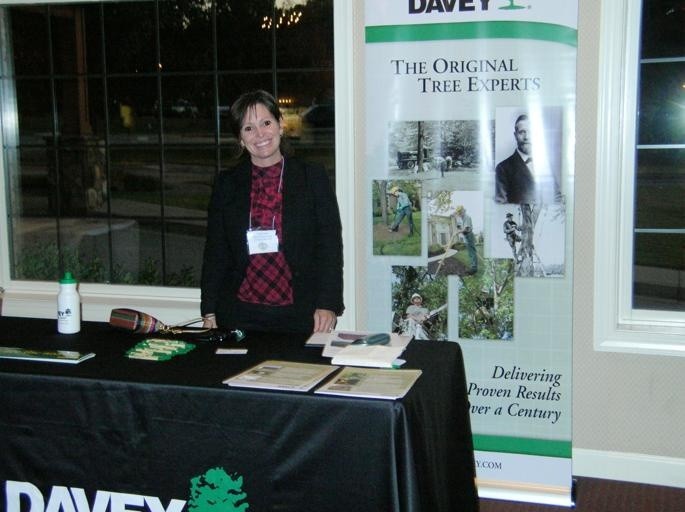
398,147 -> 434,169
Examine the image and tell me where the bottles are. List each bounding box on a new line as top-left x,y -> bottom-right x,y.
55,272 -> 81,335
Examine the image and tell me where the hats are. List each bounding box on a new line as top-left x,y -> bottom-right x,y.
411,293 -> 422,303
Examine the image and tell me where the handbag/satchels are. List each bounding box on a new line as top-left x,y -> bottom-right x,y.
110,308 -> 214,334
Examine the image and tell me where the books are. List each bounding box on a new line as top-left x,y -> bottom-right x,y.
220,359 -> 334,394
317,362 -> 424,402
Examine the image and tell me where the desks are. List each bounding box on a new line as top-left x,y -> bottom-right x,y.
0,315 -> 480,512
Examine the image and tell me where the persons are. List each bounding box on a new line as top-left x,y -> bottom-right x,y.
384,185 -> 417,239
476,280 -> 502,323
498,109 -> 561,203
196,89 -> 342,342
452,207 -> 478,274
500,214 -> 521,261
405,291 -> 432,342
